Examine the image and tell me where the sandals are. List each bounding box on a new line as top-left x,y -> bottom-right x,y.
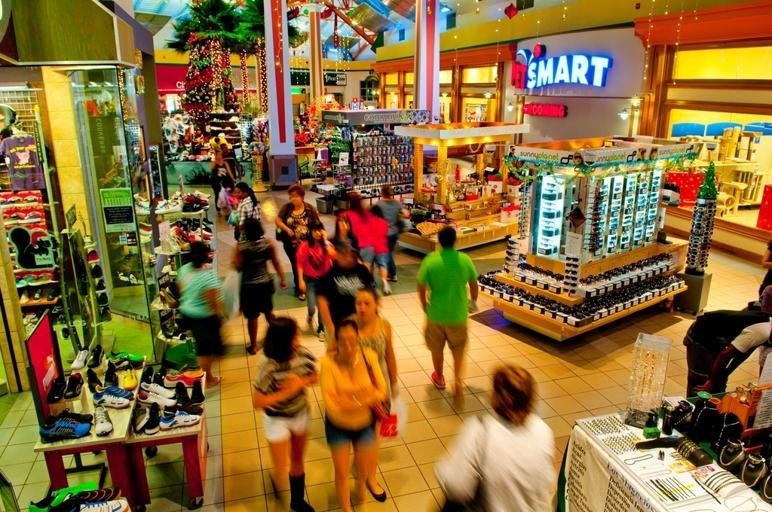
159,310 -> 175,338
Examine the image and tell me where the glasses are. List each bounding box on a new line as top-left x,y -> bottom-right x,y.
504,238 -> 519,273
477,252 -> 683,319
581,197 -> 603,252
685,202 -> 716,267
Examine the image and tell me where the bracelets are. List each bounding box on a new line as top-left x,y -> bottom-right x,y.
352,394 -> 363,409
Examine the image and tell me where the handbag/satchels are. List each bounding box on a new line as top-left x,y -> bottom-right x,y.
276,203 -> 291,240
360,345 -> 386,419
439,415 -> 488,512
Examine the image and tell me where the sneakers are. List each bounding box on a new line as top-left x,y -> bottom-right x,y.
83,234 -> 112,321
383,284 -> 393,295
29,480 -> 129,512
40,344 -> 205,443
0,195 -> 70,340
98,193 -> 158,286
154,190 -> 214,251
387,275 -> 397,281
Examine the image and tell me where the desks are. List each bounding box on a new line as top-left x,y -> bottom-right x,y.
32,368 -> 137,510
555,391 -> 772,512
123,370 -> 211,510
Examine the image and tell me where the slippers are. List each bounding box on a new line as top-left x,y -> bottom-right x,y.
247,344 -> 257,355
207,376 -> 224,387
318,330 -> 326,341
431,371 -> 446,389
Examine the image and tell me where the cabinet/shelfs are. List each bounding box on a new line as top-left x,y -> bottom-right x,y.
589,176 -> 661,255
0,166 -> 73,309
208,112 -> 241,140
314,176 -> 414,211
351,133 -> 414,175
473,245 -> 688,342
379,192 -> 517,256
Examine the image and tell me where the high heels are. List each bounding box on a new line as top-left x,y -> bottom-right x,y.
159,287 -> 179,308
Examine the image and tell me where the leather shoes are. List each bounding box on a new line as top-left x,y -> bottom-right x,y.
366,479 -> 386,501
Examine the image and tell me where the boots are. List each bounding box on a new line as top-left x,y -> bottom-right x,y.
289,471 -> 316,512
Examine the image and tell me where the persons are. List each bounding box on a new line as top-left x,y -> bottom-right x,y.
275,185 -> 406,341
231,182 -> 288,353
175,124 -> 243,215
329,286 -> 398,502
432,363 -> 558,512
758,239 -> 772,296
745,285 -> 770,311
571,145 -> 693,165
415,225 -> 479,402
320,319 -> 387,511
252,314 -> 320,512
684,309 -> 772,396
176,240 -> 231,388
